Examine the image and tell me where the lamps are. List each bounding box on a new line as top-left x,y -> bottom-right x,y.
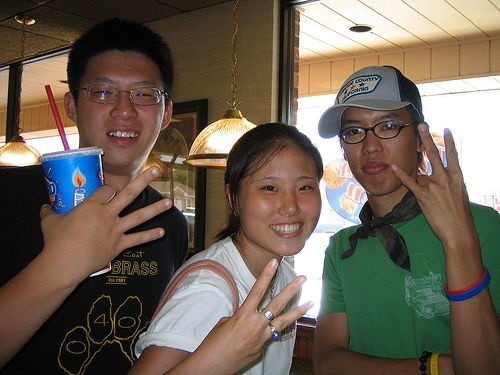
142,152 -> 168,182
182,0 -> 256,168
0,16 -> 41,167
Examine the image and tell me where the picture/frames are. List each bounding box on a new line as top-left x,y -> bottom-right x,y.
147,98 -> 206,261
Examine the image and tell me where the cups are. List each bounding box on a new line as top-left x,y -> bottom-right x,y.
40,144 -> 111,278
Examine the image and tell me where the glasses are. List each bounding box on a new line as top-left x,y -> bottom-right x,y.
339,120 -> 415,144
76,85 -> 169,106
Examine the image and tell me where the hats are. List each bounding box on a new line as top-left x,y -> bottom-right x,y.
318,65 -> 424,139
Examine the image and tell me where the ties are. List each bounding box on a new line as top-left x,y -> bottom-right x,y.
340,190 -> 421,271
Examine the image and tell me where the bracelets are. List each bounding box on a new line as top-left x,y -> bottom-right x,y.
419,351 -> 440,375
444,268 -> 490,301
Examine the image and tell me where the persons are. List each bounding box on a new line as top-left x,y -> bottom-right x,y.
128,123 -> 324,375
0,17 -> 189,375
313,65 -> 500,375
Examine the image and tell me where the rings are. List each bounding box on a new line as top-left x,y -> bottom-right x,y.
269,323 -> 280,339
262,308 -> 275,320
